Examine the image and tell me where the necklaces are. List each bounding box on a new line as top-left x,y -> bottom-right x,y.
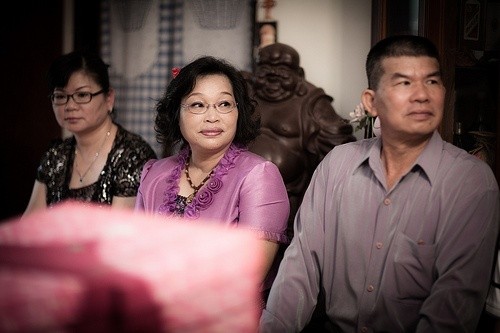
75,125 -> 112,182
185,163 -> 216,198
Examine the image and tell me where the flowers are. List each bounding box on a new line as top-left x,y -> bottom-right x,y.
348,102 -> 371,138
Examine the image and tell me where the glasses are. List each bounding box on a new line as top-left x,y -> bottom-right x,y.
181,99 -> 238,113
51,89 -> 103,104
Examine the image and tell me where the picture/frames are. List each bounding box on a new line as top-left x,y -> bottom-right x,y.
458,0 -> 487,49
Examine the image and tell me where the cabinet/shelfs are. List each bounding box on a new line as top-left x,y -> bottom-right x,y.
371,0 -> 500,167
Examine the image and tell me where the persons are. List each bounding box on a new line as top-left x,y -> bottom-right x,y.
238,42 -> 357,211
18,48 -> 155,221
134,57 -> 290,313
259,36 -> 500,333
